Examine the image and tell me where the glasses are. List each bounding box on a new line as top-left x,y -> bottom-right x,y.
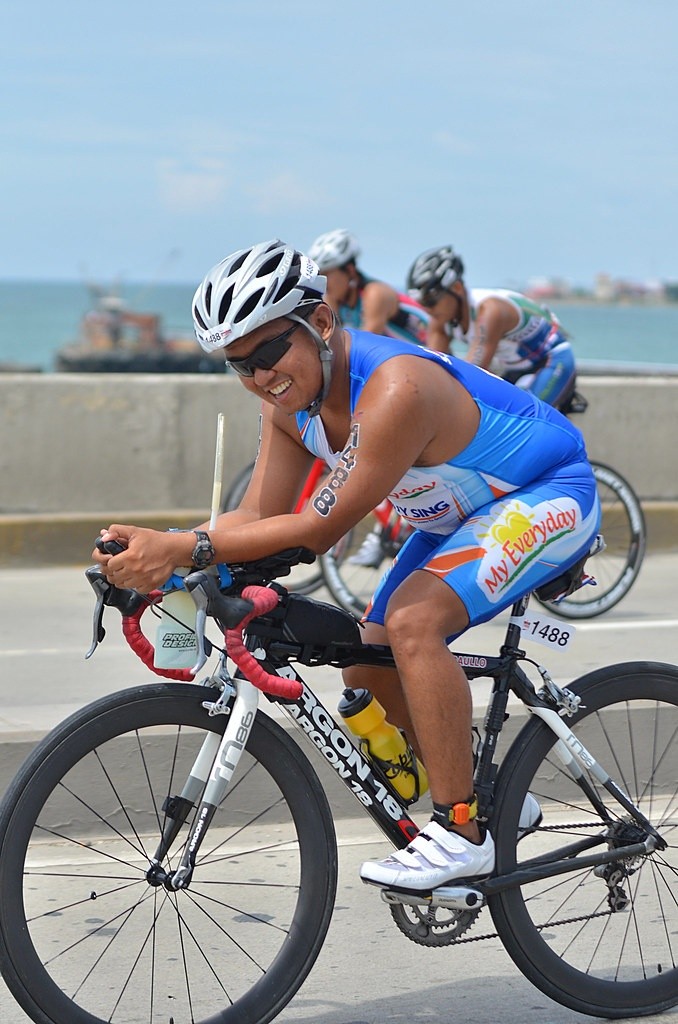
225,302 -> 319,378
417,281 -> 446,307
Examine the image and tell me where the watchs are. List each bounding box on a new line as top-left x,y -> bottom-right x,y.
190,528 -> 214,571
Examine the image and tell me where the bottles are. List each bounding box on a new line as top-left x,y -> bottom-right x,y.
151,525 -> 197,669
337,686 -> 429,802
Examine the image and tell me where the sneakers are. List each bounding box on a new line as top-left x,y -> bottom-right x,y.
360,819 -> 497,892
514,792 -> 542,841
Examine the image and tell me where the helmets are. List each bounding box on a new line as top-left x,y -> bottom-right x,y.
310,230 -> 357,271
408,246 -> 455,289
191,238 -> 320,354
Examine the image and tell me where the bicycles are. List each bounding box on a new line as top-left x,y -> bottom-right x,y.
316,388 -> 645,621
225,458 -> 352,594
1,528 -> 678,1024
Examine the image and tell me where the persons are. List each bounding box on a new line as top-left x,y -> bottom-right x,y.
90,238 -> 600,901
304,230 -> 431,348
407,242 -> 579,414
